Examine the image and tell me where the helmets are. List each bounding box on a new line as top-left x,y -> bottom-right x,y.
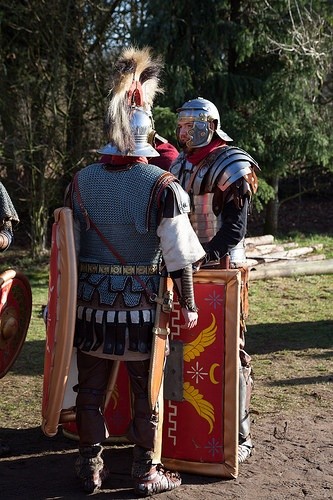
175,97 -> 234,149
96,46 -> 161,158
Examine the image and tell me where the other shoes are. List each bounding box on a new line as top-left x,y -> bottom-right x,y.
238,440 -> 255,462
83,465 -> 109,492
132,468 -> 182,495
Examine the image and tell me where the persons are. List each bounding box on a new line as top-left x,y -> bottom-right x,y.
168,96 -> 260,463
63,48 -> 207,494
